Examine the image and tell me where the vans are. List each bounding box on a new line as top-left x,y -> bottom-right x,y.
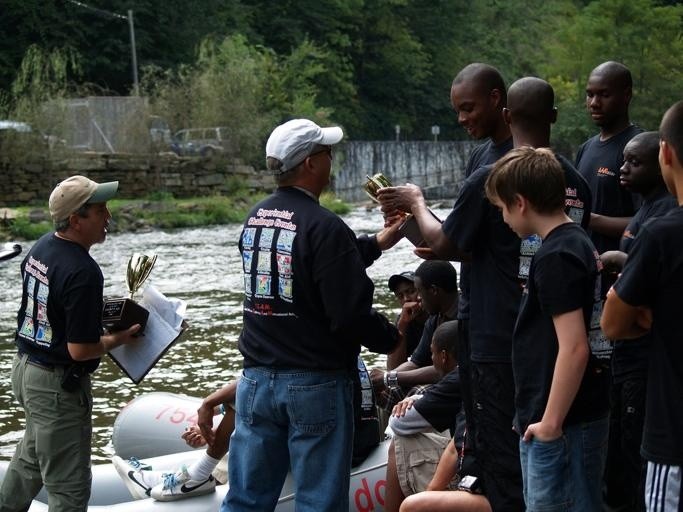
1,104 -> 241,160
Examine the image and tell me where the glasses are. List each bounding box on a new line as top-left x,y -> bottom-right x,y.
305,146 -> 334,162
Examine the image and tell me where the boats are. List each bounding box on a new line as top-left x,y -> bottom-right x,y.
108,389 -> 226,464
0,429 -> 396,512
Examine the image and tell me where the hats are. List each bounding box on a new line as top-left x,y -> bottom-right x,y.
49,175 -> 119,223
388,271 -> 416,291
265,118 -> 344,176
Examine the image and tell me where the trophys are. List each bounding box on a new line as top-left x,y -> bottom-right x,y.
125,252 -> 157,300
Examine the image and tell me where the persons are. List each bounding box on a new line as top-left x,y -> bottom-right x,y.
110,349 -> 380,502
0,175 -> 145,512
218,117 -> 403,511
360,60 -> 682,512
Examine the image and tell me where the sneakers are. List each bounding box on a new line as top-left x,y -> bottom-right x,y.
112,455 -> 153,501
151,466 -> 216,502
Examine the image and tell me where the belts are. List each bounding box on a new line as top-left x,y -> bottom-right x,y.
19,350 -> 54,368
